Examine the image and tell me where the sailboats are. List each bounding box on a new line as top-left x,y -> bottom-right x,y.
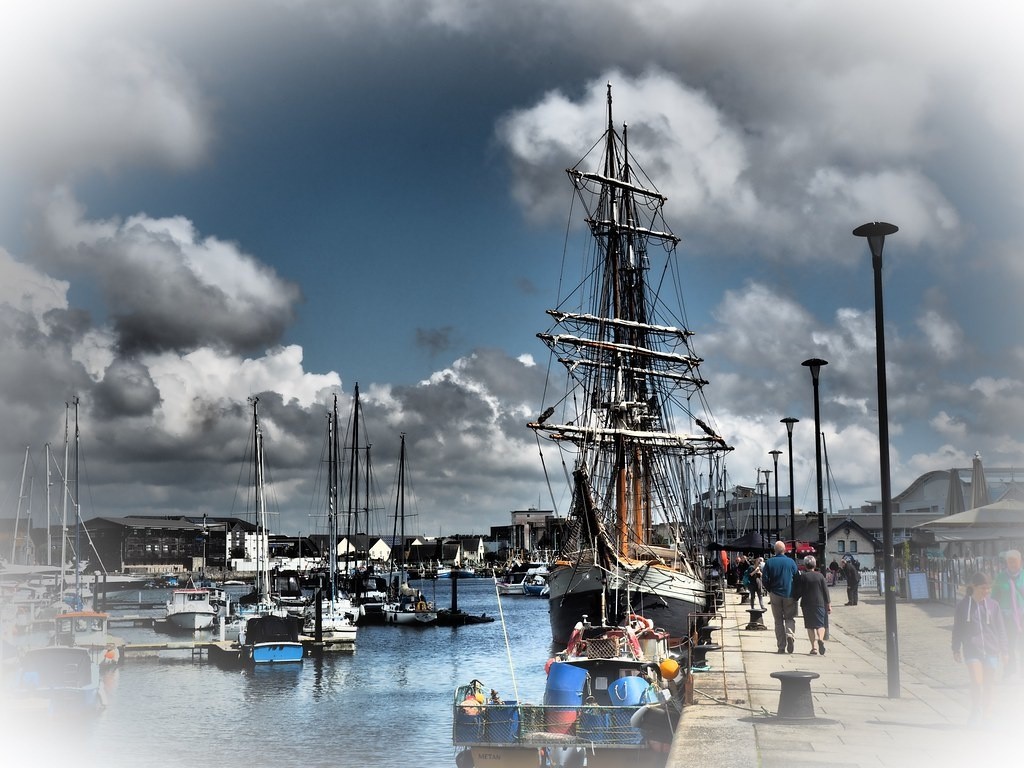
1,81 -> 735,766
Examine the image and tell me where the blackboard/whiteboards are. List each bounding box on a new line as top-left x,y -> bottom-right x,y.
877,570 -> 885,593
905,570 -> 930,600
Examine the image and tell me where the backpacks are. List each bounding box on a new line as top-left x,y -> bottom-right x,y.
742,566 -> 754,585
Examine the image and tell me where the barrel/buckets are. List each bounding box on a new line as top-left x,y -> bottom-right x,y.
543,661 -> 657,743
456,713 -> 486,743
486,700 -> 519,743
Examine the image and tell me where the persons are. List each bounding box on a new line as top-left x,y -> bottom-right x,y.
951,571 -> 1009,691
841,559 -> 861,606
713,555 -> 765,609
830,558 -> 839,585
762,541 -> 800,654
795,555 -> 832,655
992,550 -> 1024,680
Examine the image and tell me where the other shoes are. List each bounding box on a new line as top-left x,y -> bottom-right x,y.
810,648 -> 817,654
778,648 -> 785,654
786,637 -> 793,653
818,640 -> 825,655
844,602 -> 857,606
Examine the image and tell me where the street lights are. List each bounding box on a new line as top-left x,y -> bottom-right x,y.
768,449 -> 783,543
780,416 -> 800,564
852,222 -> 904,700
761,470 -> 773,559
802,358 -> 830,581
756,482 -> 767,560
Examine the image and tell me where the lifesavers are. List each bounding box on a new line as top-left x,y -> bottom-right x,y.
417,601 -> 426,610
625,614 -> 650,632
566,621 -> 583,655
213,603 -> 218,612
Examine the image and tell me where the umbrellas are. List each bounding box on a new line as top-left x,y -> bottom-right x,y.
911,498 -> 1024,541
943,468 -> 966,558
970,458 -> 988,512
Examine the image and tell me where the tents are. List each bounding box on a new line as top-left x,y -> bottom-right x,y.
784,542 -> 815,553
723,532 -> 775,554
